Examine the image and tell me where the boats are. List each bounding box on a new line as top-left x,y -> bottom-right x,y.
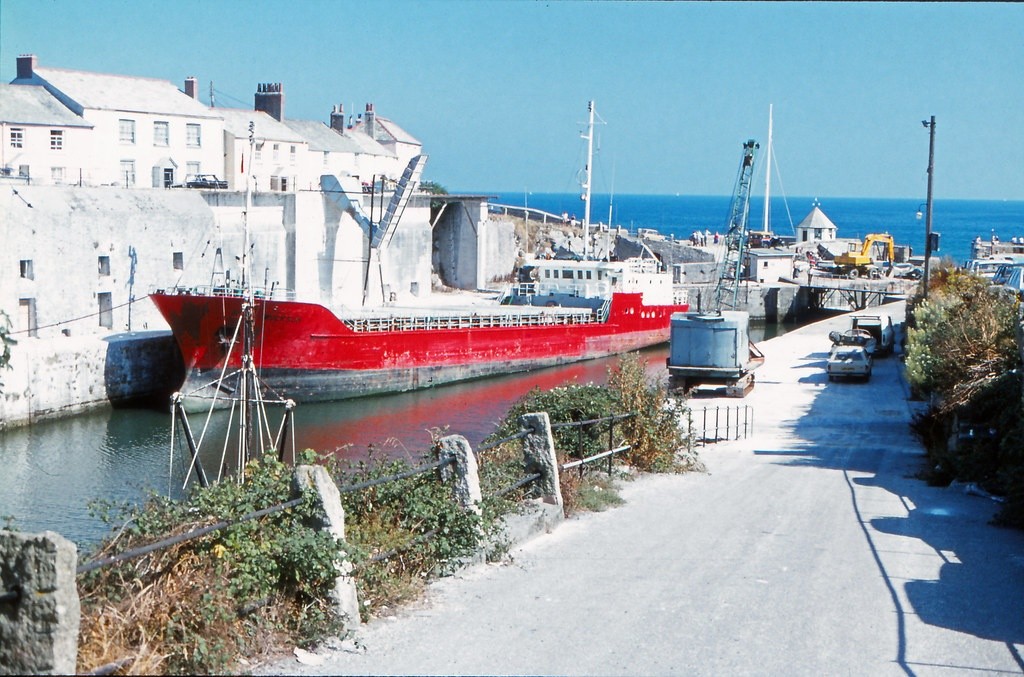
144,99 -> 690,415
960,228 -> 1024,303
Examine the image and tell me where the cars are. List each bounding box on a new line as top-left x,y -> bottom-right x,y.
824,328 -> 878,383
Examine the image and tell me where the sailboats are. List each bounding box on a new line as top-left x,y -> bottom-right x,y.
724,102 -> 797,250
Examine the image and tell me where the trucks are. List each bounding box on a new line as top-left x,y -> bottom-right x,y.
848,314 -> 883,355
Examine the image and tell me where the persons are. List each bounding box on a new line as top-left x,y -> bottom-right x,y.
808,266 -> 815,284
691,230 -> 719,248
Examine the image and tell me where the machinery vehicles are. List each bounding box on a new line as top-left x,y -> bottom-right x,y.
666,138 -> 769,399
830,229 -> 895,279
